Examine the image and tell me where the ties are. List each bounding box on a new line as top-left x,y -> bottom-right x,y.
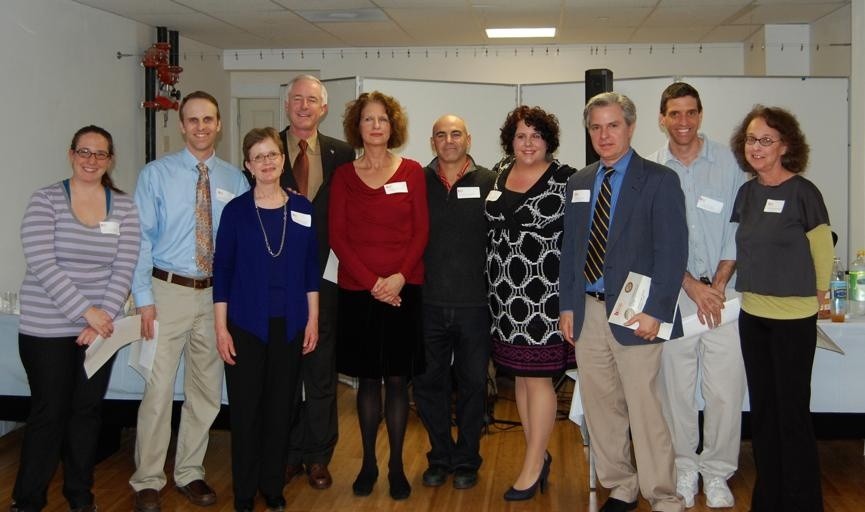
584,167 -> 615,285
195,163 -> 213,276
292,140 -> 309,199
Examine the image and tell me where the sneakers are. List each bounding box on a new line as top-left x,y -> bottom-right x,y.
675,475 -> 698,509
705,480 -> 733,508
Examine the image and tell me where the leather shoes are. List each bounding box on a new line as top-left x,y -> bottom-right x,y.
454,470 -> 477,487
134,489 -> 160,511
234,495 -> 254,512
285,465 -> 304,480
10,501 -> 22,512
599,497 -> 639,512
353,467 -> 378,496
70,494 -> 96,511
423,464 -> 447,485
178,480 -> 215,506
264,494 -> 285,508
388,468 -> 411,499
310,464 -> 332,488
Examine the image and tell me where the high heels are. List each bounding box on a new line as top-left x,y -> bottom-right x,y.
504,459 -> 548,500
546,451 -> 553,467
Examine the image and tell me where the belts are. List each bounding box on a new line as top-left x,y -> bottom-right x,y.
588,290 -> 603,303
152,268 -> 213,288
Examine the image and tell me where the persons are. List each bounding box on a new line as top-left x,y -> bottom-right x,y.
731,108 -> 838,512
558,93 -> 690,512
12,124 -> 141,511
214,128 -> 321,512
486,107 -> 579,500
419,115 -> 498,488
636,82 -> 747,509
328,92 -> 428,499
129,92 -> 304,512
244,75 -> 355,487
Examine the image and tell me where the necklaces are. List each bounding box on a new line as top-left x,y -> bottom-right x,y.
253,194 -> 287,258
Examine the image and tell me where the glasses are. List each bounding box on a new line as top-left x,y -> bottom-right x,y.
744,136 -> 782,146
72,149 -> 111,159
247,152 -> 282,161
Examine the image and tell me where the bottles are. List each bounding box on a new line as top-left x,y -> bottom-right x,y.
848,250 -> 865,319
829,258 -> 848,321
817,289 -> 831,319
